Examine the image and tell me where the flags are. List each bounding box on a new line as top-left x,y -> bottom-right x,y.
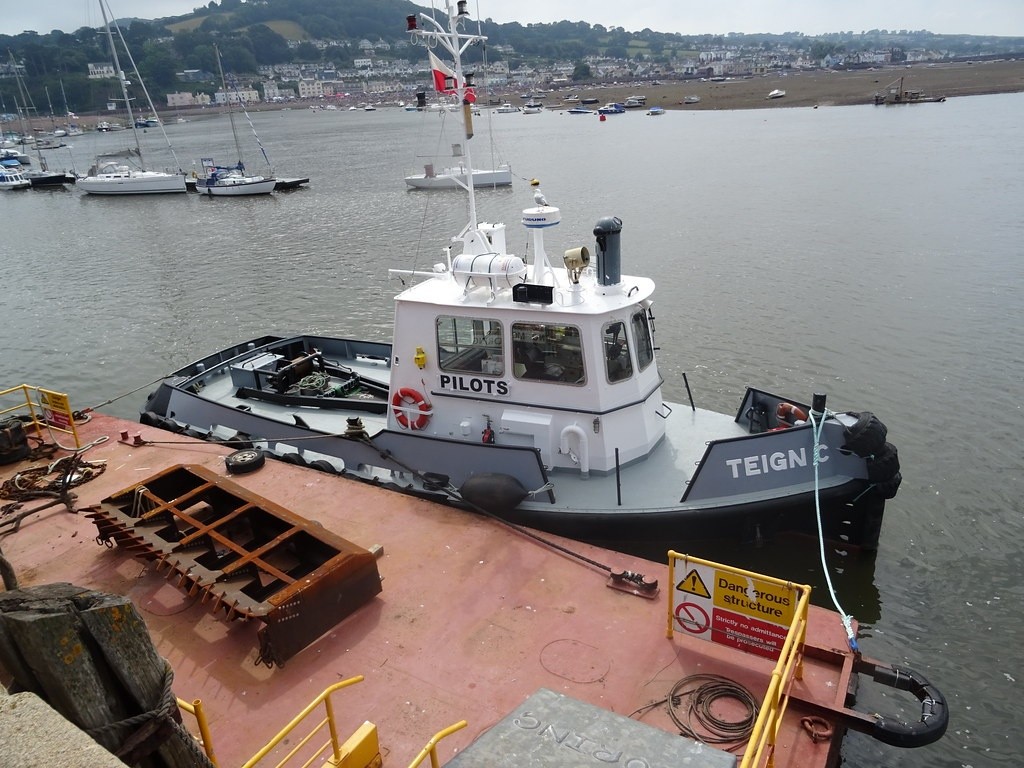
428,50 -> 475,103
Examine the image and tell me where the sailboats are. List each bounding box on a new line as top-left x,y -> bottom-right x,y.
75,0 -> 188,195
0,45 -> 184,193
191,41 -> 279,196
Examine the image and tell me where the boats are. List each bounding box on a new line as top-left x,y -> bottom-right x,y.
404,44 -> 513,188
136,0 -> 903,590
252,58 -> 1023,119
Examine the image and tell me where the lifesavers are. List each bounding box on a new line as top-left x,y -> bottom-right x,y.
777,403 -> 807,428
393,388 -> 428,430
